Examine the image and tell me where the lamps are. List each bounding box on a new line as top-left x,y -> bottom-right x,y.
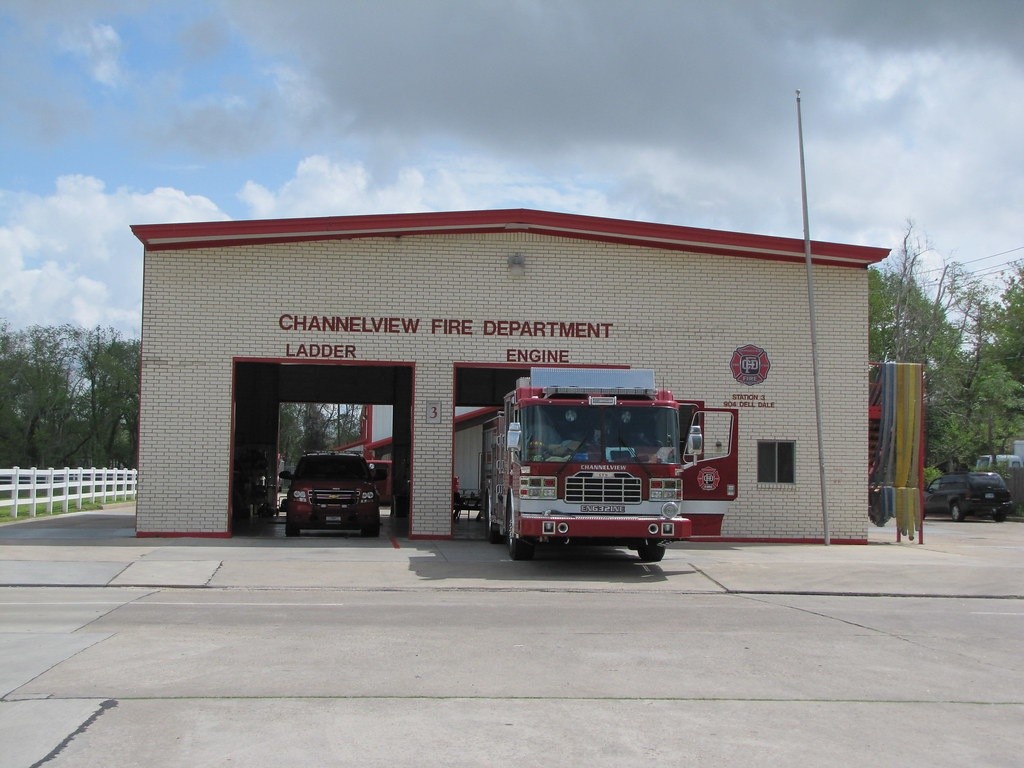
507,253 -> 525,275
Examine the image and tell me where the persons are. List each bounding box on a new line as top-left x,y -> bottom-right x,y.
256,480 -> 266,515
453,475 -> 461,521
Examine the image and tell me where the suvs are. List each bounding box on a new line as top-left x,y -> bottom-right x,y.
924,470 -> 1014,523
278,451 -> 382,536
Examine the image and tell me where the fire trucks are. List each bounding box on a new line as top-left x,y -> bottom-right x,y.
479,366 -> 742,566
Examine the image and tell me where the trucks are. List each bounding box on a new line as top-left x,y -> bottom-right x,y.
367,460 -> 459,505
